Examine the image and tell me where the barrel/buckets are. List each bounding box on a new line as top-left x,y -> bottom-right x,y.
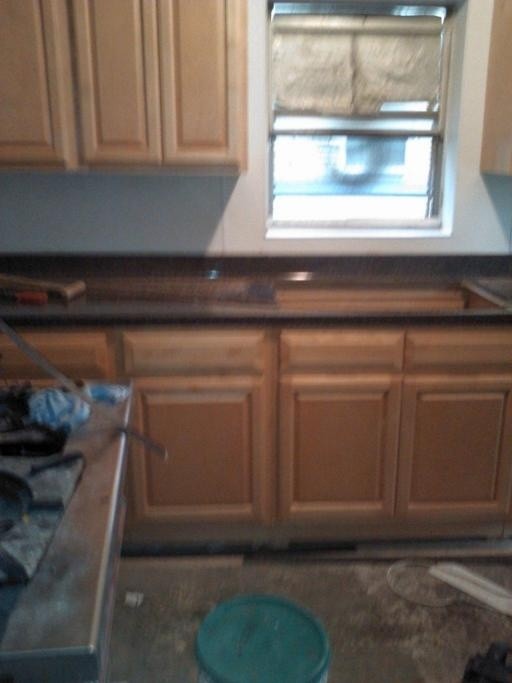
196,589 -> 329,683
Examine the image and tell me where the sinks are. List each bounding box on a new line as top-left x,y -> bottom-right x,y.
269,277 -> 511,319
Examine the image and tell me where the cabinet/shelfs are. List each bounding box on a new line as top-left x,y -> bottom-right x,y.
2,0 -> 71,173
72,1 -> 246,175
0,330 -> 113,385
122,328 -> 271,556
